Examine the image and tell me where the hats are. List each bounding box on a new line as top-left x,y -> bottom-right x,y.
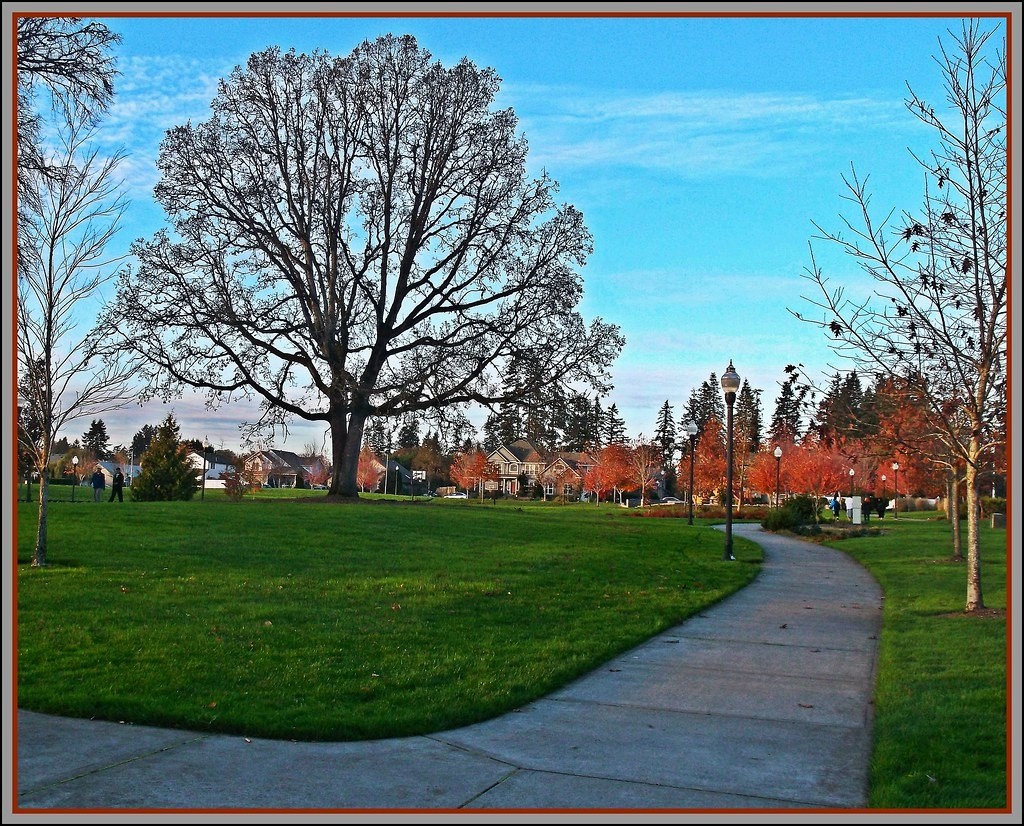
97,465 -> 102,470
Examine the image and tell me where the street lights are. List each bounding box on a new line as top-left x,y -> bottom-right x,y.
881,474 -> 887,498
774,444 -> 782,511
892,460 -> 899,519
687,420 -> 698,526
849,469 -> 855,495
721,359 -> 740,566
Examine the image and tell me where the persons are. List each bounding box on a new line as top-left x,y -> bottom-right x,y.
108,467 -> 124,503
90,465 -> 105,502
806,492 -> 885,521
